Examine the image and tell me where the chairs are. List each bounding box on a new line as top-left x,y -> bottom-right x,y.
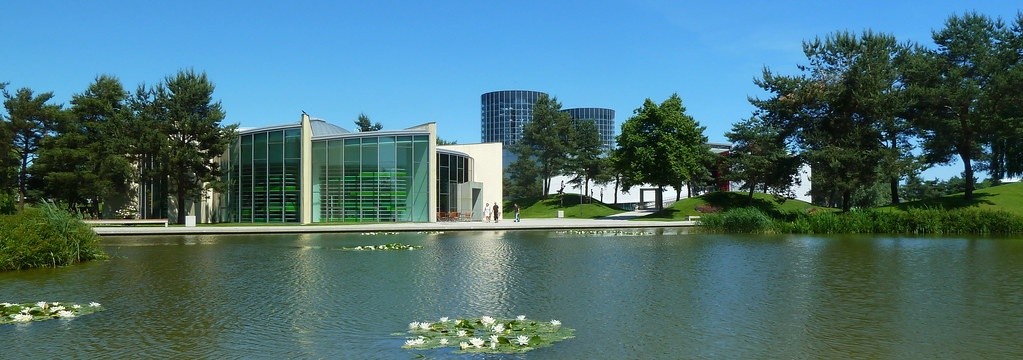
437,211 -> 473,222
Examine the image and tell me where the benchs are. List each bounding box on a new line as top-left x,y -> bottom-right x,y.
689,215 -> 700,221
84,218 -> 169,227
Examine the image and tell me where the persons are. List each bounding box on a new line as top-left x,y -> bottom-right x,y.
513,203 -> 521,222
483,203 -> 492,223
491,202 -> 499,223
560,180 -> 566,194
